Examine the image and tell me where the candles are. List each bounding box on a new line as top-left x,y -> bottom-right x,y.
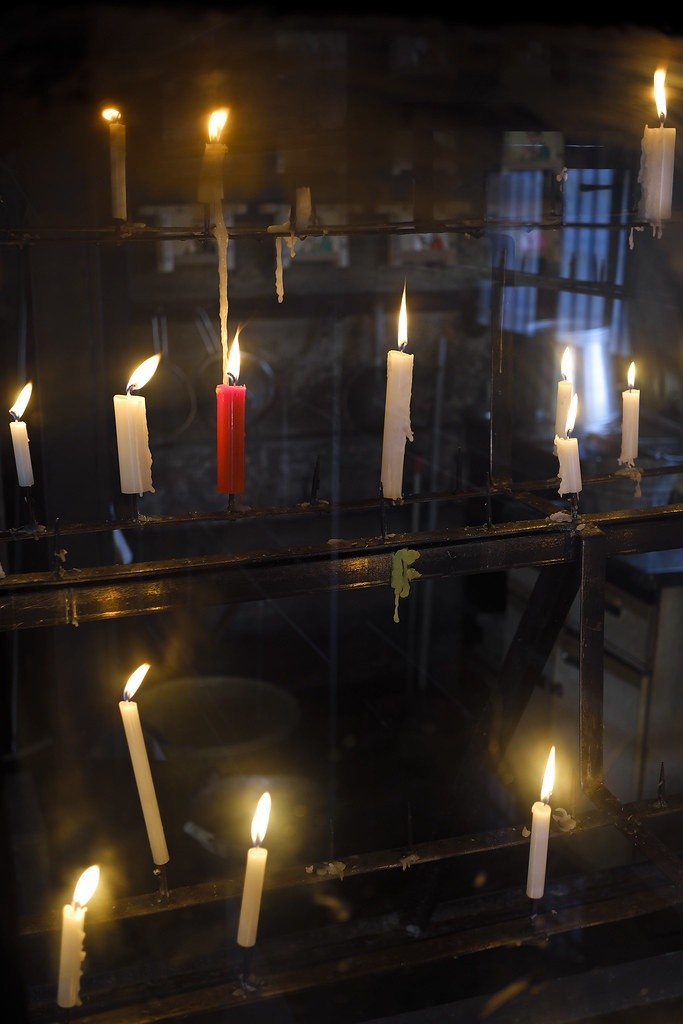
9,381 -> 35,487
57,865 -> 100,1008
381,284 -> 413,498
526,745 -> 556,899
637,68 -> 676,220
621,361 -> 640,460
296,184 -> 312,225
103,108 -> 126,220
555,346 -> 572,437
554,394 -> 582,494
216,325 -> 246,494
119,663 -> 169,865
237,792 -> 271,947
113,354 -> 160,493
198,110 -> 227,202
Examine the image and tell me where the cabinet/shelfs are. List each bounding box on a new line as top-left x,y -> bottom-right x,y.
500,566 -> 682,866
